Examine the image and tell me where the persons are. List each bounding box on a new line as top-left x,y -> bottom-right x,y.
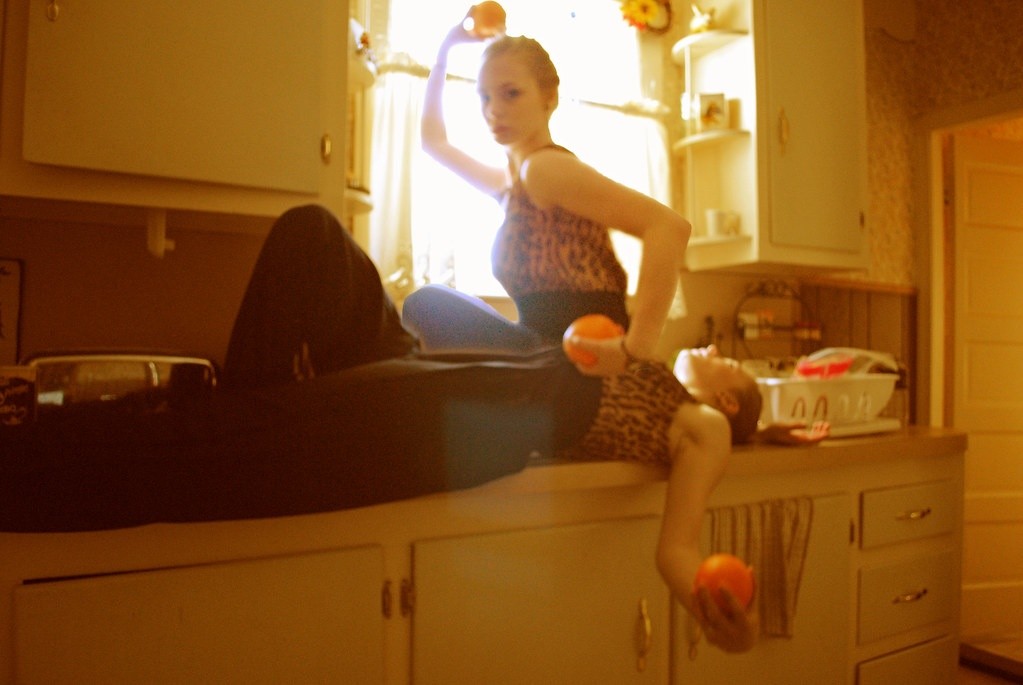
403,5 -> 692,377
0,204 -> 831,656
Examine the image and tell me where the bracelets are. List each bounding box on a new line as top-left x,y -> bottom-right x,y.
619,340 -> 647,365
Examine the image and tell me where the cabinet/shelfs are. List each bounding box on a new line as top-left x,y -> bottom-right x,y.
1,1 -> 349,224
1,452 -> 964,684
669,1 -> 875,273
